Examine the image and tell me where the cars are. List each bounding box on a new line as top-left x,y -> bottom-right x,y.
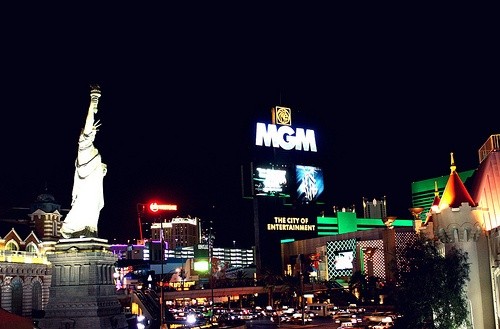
169,304 -> 401,329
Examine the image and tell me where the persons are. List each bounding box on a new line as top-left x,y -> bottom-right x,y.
59,85 -> 107,239
